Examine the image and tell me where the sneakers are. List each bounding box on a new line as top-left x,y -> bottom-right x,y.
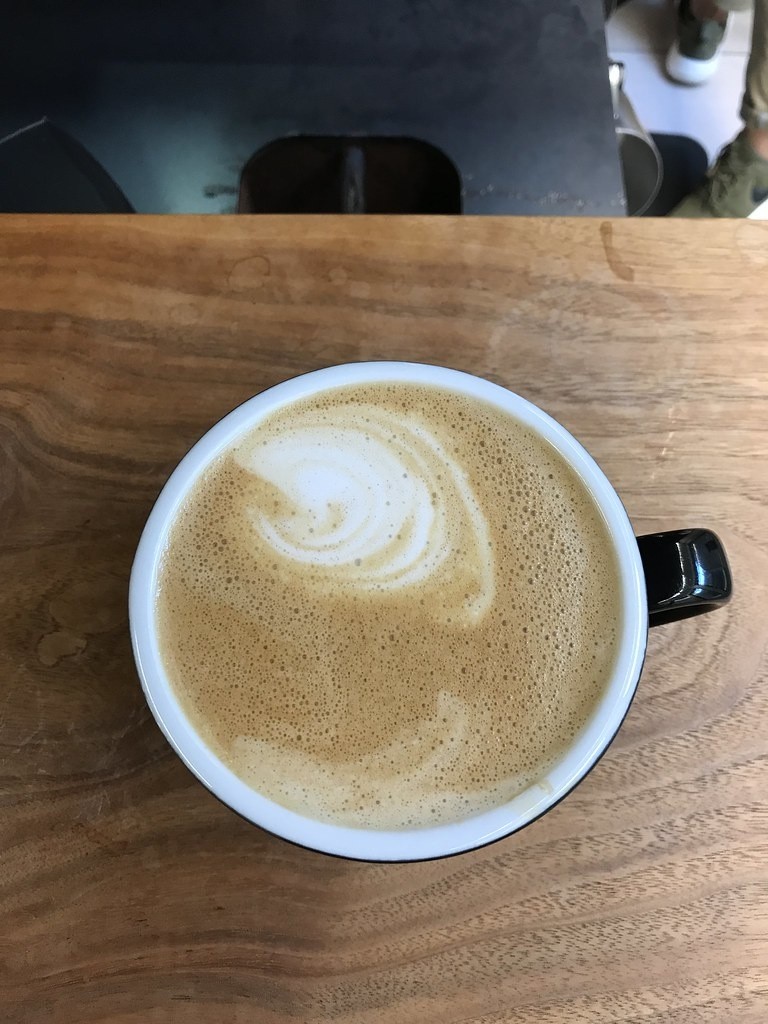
669,129 -> 768,219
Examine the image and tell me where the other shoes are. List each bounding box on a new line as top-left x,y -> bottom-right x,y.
664,10 -> 734,85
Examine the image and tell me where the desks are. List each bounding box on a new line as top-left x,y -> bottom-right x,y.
0,214 -> 768,1024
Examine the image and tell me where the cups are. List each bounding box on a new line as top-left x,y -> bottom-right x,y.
129,361 -> 734,863
